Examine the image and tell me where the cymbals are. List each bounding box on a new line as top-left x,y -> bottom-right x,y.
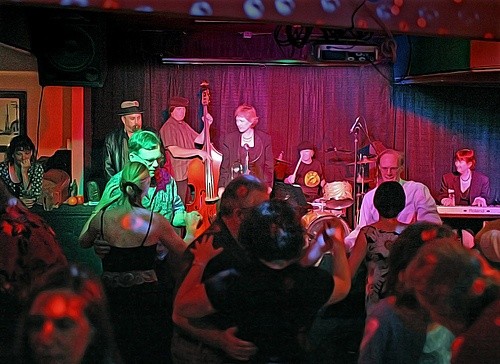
344,178 -> 374,182
345,159 -> 377,165
274,157 -> 293,165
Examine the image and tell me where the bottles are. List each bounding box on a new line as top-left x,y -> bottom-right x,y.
448,190 -> 456,206
71,179 -> 77,196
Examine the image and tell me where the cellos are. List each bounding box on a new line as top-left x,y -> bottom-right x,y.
185,81 -> 223,238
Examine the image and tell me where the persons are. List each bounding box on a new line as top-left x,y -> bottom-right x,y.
348,180 -> 412,323
169,174 -> 333,364
358,219 -> 500,364
284,142 -> 328,204
160,96 -> 213,208
8,265 -> 113,364
79,131 -> 203,364
343,150 -> 443,252
175,200 -> 351,364
436,149 -> 490,249
0,134 -> 44,210
218,103 -> 274,200
103,100 -> 167,183
0,181 -> 75,364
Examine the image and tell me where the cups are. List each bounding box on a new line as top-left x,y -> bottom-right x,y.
42,191 -> 54,211
75,195 -> 84,204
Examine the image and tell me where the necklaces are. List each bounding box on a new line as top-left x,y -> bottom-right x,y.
460,175 -> 471,182
243,135 -> 253,142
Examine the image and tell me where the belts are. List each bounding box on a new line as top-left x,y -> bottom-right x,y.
173,328 -> 226,356
101,269 -> 158,288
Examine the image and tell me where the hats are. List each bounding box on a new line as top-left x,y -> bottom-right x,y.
117,101 -> 144,116
168,97 -> 189,107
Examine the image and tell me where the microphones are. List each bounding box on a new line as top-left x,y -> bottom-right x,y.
350,116 -> 361,134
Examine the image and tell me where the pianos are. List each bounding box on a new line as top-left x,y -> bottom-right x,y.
437,205 -> 500,218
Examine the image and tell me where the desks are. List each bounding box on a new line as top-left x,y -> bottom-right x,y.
26,202 -> 102,276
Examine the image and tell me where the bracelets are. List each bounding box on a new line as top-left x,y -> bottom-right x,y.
194,261 -> 206,268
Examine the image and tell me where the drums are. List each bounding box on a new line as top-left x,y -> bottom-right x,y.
302,210 -> 351,242
322,180 -> 354,209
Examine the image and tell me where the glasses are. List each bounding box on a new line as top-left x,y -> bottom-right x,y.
132,152 -> 163,166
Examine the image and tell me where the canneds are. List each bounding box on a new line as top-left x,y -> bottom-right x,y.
448,194 -> 455,206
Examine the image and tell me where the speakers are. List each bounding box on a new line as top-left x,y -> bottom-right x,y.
36,10 -> 108,88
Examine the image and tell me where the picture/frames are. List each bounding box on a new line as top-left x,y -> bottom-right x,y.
0,90 -> 27,153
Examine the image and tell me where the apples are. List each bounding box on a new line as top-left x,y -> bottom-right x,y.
69,195 -> 84,205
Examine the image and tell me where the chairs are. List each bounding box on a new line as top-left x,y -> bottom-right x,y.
42,169 -> 69,207
38,148 -> 72,179
